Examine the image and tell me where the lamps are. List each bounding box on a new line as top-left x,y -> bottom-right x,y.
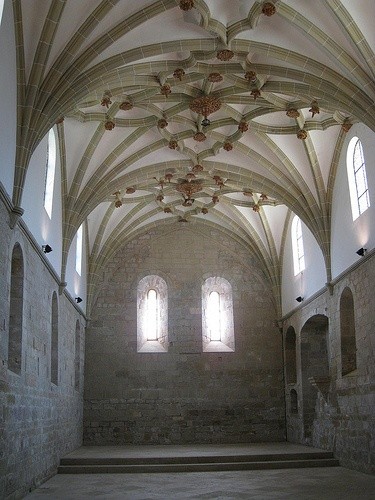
357,247 -> 366,256
75,296 -> 82,304
41,243 -> 52,254
296,295 -> 304,302
